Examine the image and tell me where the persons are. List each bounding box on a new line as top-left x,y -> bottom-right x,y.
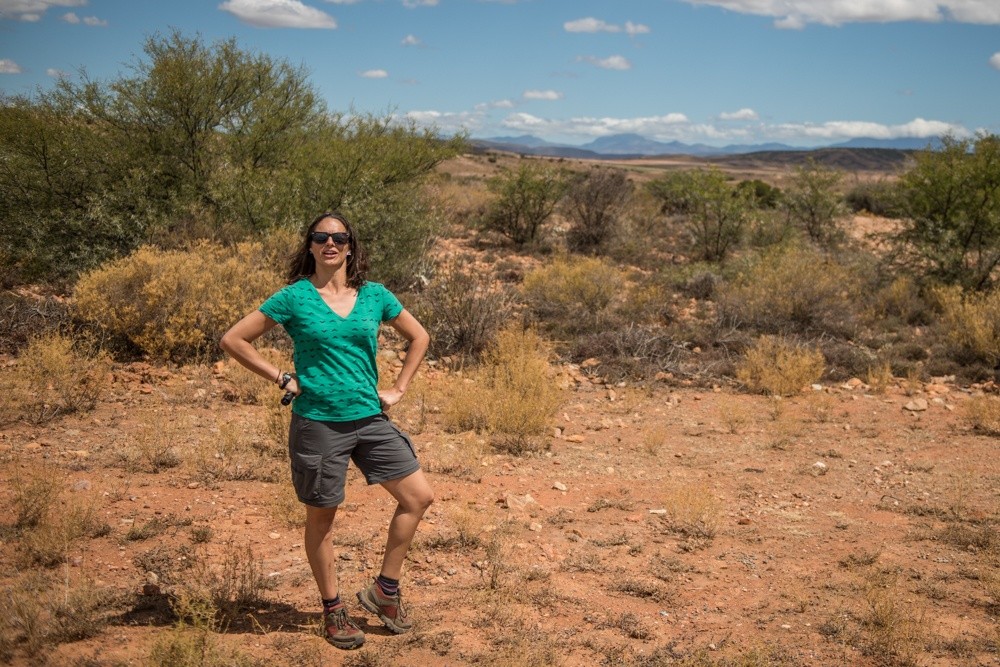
220,212 -> 435,650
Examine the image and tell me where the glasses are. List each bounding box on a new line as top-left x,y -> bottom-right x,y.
309,231 -> 351,245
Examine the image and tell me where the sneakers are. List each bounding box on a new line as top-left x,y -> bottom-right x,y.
355,575 -> 412,635
323,601 -> 367,650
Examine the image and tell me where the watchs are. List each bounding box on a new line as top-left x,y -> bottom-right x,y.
279,372 -> 292,390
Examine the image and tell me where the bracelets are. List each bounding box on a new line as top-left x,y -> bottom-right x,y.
274,369 -> 282,384
